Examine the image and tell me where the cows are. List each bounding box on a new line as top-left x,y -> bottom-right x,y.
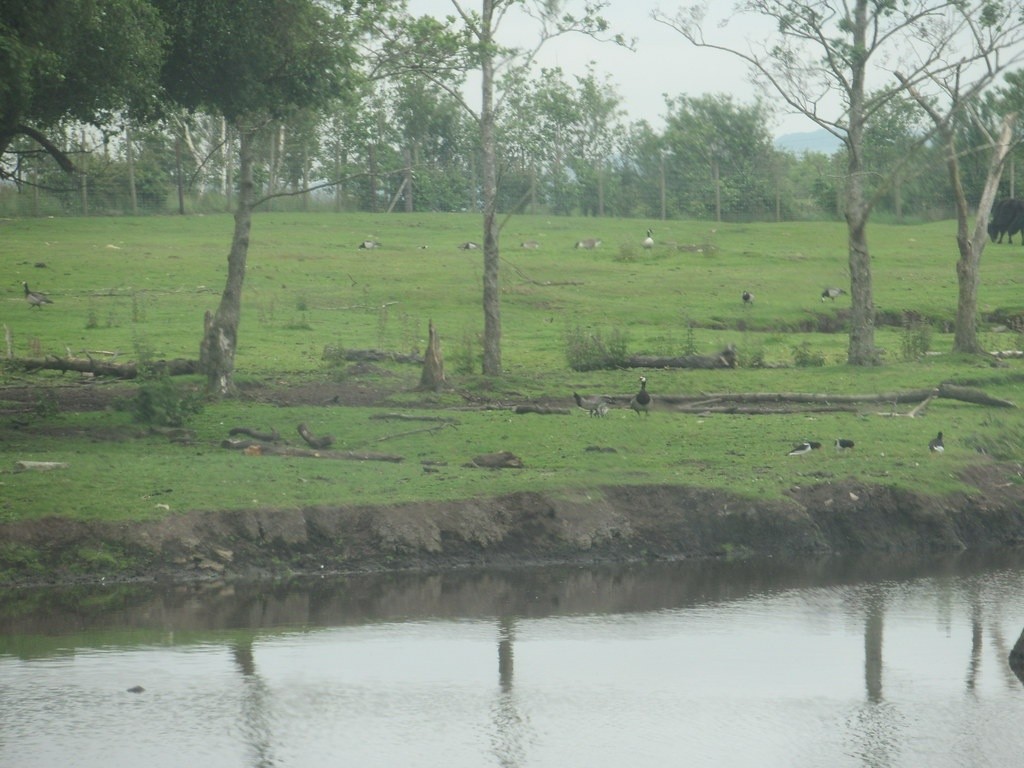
988,199 -> 1024,246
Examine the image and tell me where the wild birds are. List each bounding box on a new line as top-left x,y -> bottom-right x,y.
458,242 -> 480,251
22,282 -> 53,309
742,290 -> 755,305
821,287 -> 850,303
833,439 -> 855,450
571,391 -> 617,418
784,442 -> 821,457
631,376 -> 652,416
359,240 -> 383,250
928,431 -> 946,453
639,228 -> 655,248
